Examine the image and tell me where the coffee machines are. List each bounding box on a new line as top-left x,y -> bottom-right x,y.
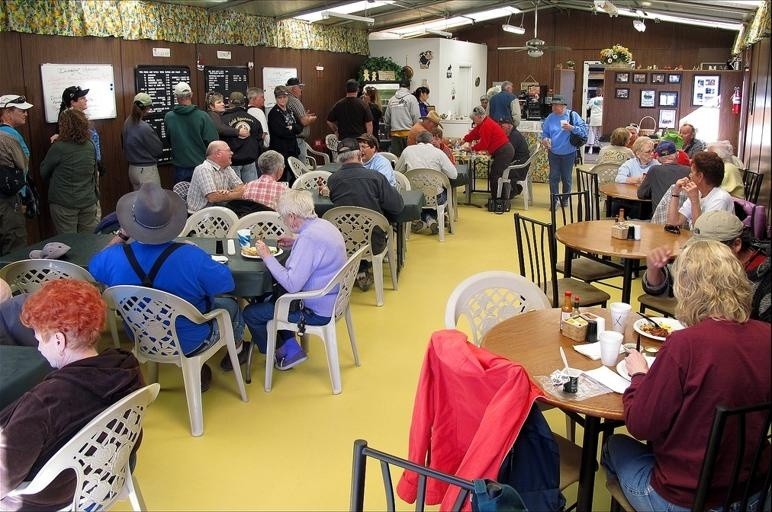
527,84 -> 548,120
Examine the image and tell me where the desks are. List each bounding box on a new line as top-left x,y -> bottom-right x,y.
1,233 -> 293,385
554,219 -> 695,306
480,306 -> 692,511
1,345 -> 58,412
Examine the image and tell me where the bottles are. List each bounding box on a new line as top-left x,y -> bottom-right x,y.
561,290 -> 574,334
627,224 -> 641,241
453,112 -> 457,120
615,207 -> 624,225
570,294 -> 580,316
227,239 -> 236,255
589,316 -> 605,341
448,139 -> 479,156
216,240 -> 223,254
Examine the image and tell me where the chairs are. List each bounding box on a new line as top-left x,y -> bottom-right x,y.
443,270 -> 575,442
172,138 -> 471,308
432,328 -> 600,511
573,137 -> 772,240
692,401 -> 771,511
264,244 -> 372,395
0,380 -> 162,511
102,284 -> 250,437
637,290 -> 679,320
551,189 -> 627,287
349,439 -> 502,512
515,212 -> 611,309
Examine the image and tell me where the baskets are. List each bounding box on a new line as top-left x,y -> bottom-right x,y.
639,116 -> 657,136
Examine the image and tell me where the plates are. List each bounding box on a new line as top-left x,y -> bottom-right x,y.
240,246 -> 283,260
633,315 -> 684,343
210,254 -> 229,267
617,356 -> 655,382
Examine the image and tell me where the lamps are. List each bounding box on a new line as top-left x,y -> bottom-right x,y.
320,6 -> 526,38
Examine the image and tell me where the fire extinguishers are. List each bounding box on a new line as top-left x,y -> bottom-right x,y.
729,87 -> 742,115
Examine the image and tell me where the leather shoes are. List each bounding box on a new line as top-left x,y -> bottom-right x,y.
201,363 -> 211,393
221,341 -> 251,372
357,272 -> 374,291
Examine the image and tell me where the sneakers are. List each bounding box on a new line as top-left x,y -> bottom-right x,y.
562,199 -> 568,206
275,350 -> 308,371
550,202 -> 558,211
411,220 -> 427,234
427,218 -> 439,235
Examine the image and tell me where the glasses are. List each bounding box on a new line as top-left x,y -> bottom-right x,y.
73,86 -> 81,93
473,107 -> 481,115
207,92 -> 214,99
19,109 -> 26,113
627,123 -> 637,127
361,144 -> 370,149
278,214 -> 283,220
4,94 -> 26,110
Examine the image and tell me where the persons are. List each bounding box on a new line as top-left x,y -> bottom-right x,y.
382,78 -> 458,234
1,86 -> 101,254
458,81 -> 530,206
88,183 -> 253,392
165,78 -> 317,217
600,240 -> 770,511
243,189 -> 348,371
326,79 -> 404,291
586,86 -> 605,153
597,123 -> 772,318
121,92 -> 163,191
540,94 -> 589,210
1,279 -> 146,512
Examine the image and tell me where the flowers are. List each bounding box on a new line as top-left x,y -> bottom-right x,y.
599,43 -> 633,65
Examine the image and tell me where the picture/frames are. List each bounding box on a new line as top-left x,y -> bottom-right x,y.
614,70 -> 682,130
691,73 -> 722,107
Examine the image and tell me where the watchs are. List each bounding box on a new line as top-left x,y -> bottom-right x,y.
115,230 -> 129,241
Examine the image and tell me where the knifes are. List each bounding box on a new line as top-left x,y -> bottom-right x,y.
628,334 -> 641,376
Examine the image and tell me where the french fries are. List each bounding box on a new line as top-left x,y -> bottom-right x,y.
241,246 -> 259,255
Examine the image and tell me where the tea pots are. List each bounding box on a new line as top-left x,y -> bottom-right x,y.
440,114 -> 448,119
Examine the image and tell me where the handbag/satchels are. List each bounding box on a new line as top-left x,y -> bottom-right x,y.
270,285 -> 305,338
570,110 -> 588,147
488,198 -> 511,214
20,172 -> 40,219
0,164 -> 35,207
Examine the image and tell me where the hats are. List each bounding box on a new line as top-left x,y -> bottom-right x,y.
62,85 -> 89,100
400,80 -> 410,88
419,111 -> 443,129
682,210 -> 744,251
356,132 -> 379,150
115,181 -> 187,244
0,94 -> 33,110
229,91 -> 244,105
172,81 -> 191,95
337,138 -> 360,155
286,78 -> 305,87
133,92 -> 154,107
656,140 -> 676,156
550,94 -> 567,106
472,477 -> 529,512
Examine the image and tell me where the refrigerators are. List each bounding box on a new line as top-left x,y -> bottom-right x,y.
360,79 -> 411,151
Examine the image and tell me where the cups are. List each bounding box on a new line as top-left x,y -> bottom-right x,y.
560,120 -> 567,130
561,368 -> 583,394
599,331 -> 625,367
644,345 -> 658,356
236,229 -> 252,249
610,302 -> 632,336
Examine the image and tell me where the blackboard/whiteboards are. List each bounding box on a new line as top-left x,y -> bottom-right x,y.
40,62 -> 117,123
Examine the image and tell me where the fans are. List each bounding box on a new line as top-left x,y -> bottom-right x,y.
497,0 -> 574,58
